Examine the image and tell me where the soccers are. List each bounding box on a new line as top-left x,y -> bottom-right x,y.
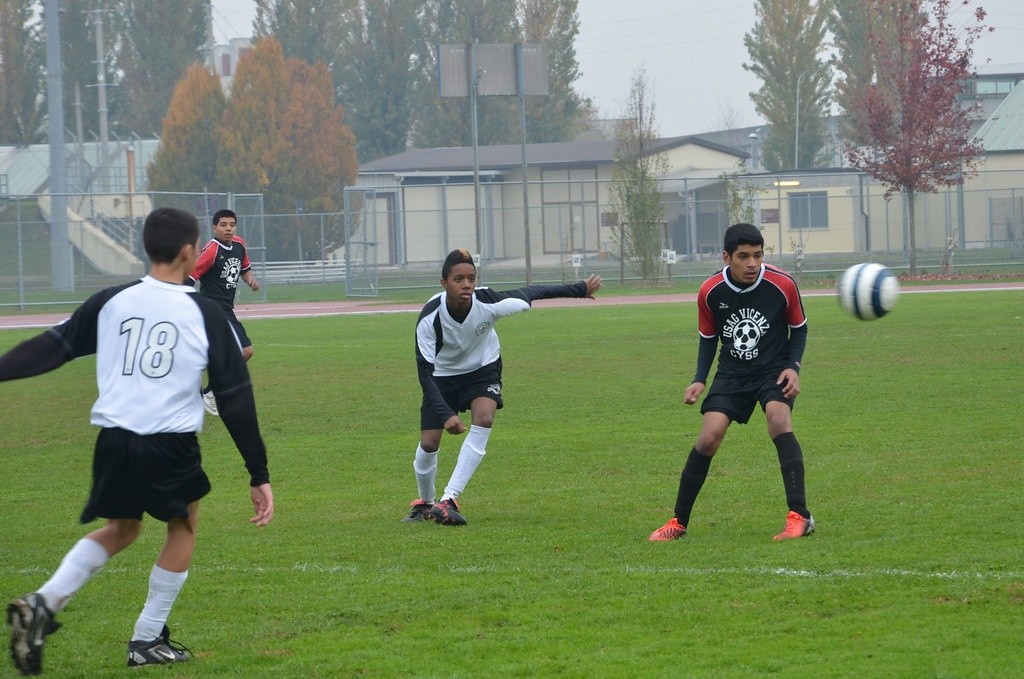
837,262 -> 901,321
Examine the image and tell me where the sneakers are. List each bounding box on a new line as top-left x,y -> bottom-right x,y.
399,498 -> 432,523
427,498 -> 467,526
648,518 -> 687,541
770,511 -> 816,541
5,593 -> 61,675
202,391 -> 219,416
127,624 -> 197,667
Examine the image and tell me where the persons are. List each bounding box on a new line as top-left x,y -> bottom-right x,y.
400,250 -> 602,525
0,207 -> 274,675
183,209 -> 260,416
649,224 -> 814,542
